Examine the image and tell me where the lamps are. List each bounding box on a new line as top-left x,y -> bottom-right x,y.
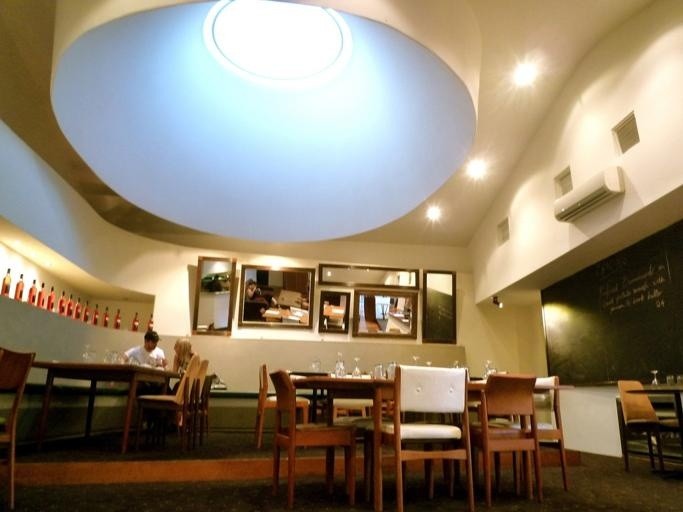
493,296 -> 503,309
204,0 -> 353,92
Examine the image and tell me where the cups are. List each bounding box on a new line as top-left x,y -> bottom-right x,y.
651,370 -> 659,386
352,358 -> 363,377
388,361 -> 396,382
373,363 -> 382,381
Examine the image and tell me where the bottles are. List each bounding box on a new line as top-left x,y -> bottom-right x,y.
59,291 -> 66,316
147,313 -> 152,332
47,286 -> 54,313
66,294 -> 74,318
29,279 -> 38,307
115,308 -> 122,330
83,299 -> 90,323
1,268 -> 12,298
14,273 -> 25,301
133,311 -> 139,332
103,306 -> 109,328
93,303 -> 99,326
73,296 -> 82,319
38,282 -> 46,309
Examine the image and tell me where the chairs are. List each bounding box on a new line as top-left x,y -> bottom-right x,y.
363,363 -> 475,511
472,374 -> 537,508
135,354 -> 200,454
192,360 -> 210,453
199,374 -> 219,446
616,380 -> 680,473
255,364 -> 309,449
0,346 -> 37,510
269,371 -> 356,510
537,376 -> 570,493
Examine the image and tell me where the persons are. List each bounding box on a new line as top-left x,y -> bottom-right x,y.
170,340 -> 197,428
121,332 -> 173,397
205,274 -> 222,292
260,294 -> 277,314
246,281 -> 262,298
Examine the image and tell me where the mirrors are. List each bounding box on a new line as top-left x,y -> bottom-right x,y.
352,288 -> 418,340
238,264 -> 316,330
193,255 -> 238,336
423,268 -> 457,344
318,262 -> 420,291
319,289 -> 350,334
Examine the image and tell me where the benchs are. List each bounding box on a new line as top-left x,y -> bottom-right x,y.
0,383 -> 124,443
123,390 -> 312,433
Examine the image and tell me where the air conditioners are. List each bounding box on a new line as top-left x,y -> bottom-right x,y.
553,166 -> 626,222
